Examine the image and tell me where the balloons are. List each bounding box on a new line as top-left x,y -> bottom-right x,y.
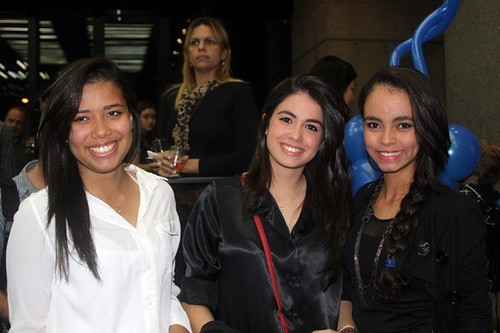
346,0 -> 480,194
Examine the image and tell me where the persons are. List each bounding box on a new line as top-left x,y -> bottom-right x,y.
128,103 -> 160,175
152,16 -> 264,225
174,75 -> 353,332
338,65 -> 500,333
305,53 -> 359,109
0,105 -> 47,333
5,58 -> 192,332
460,136 -> 500,333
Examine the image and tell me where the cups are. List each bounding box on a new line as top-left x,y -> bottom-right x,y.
167,146 -> 188,178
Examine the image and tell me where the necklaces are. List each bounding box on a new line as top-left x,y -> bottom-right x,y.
82,175 -> 134,215
272,175 -> 308,214
352,170 -> 416,301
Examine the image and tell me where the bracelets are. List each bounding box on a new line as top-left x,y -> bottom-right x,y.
337,325 -> 360,333
